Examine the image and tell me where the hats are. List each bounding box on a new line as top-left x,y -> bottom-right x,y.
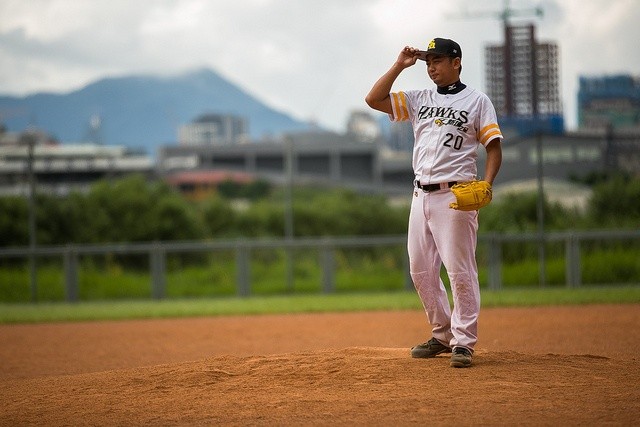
414,38 -> 462,61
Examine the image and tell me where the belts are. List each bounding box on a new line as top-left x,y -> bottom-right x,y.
417,181 -> 456,193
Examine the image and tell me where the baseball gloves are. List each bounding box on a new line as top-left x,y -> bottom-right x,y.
449,180 -> 493,211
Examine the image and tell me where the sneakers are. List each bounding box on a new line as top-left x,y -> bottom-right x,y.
450,347 -> 473,368
411,338 -> 453,359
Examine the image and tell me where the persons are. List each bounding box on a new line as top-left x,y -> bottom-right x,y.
365,35 -> 502,368
